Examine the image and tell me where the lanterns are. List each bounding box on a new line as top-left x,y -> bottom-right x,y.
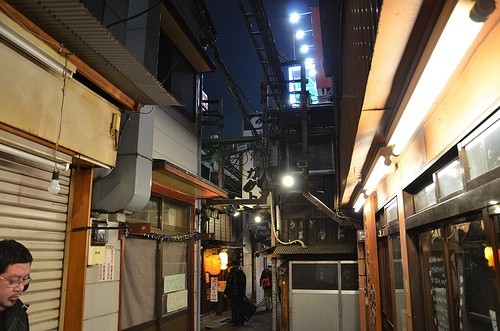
208,252 -> 228,275
484,247 -> 495,266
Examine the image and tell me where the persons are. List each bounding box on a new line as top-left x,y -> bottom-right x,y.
224,260 -> 246,326
0,239 -> 33,331
260,265 -> 281,311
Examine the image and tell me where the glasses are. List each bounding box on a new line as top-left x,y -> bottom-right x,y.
0,275 -> 32,287
